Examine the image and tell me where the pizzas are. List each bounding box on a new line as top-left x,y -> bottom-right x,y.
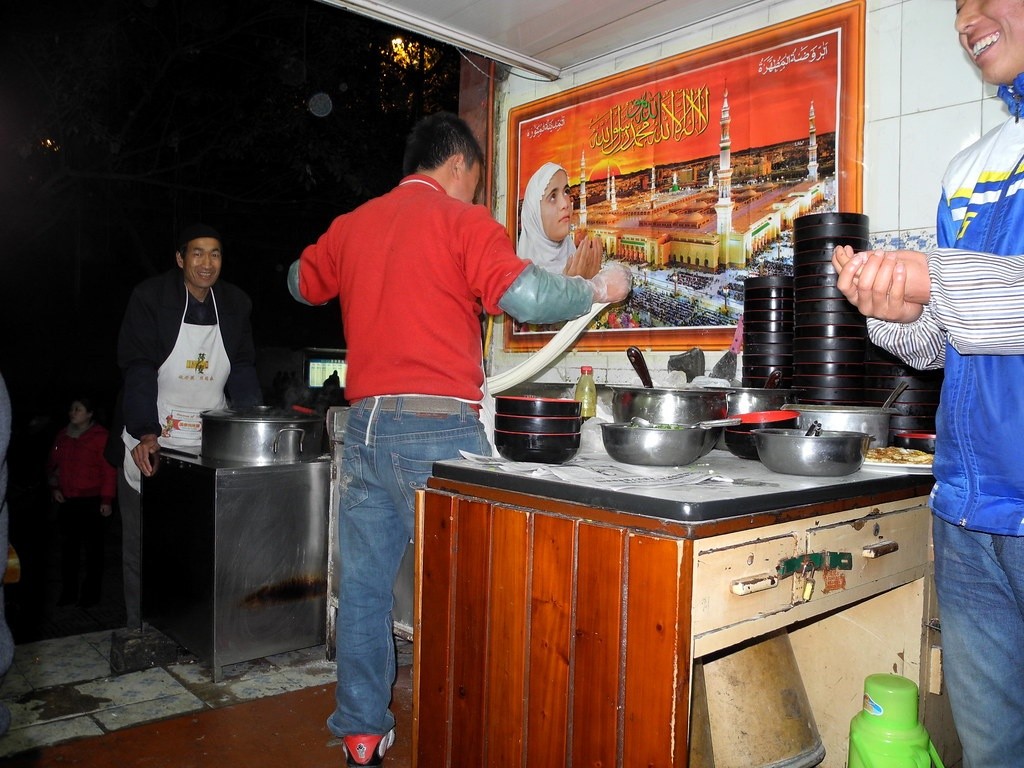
867,446 -> 934,465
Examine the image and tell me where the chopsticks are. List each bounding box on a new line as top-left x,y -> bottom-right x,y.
805,420 -> 822,438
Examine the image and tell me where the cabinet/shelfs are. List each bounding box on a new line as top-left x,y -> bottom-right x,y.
409,444 -> 963,768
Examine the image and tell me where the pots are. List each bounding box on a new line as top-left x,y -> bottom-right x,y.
199,401 -> 327,462
605,384 -> 735,457
704,387 -> 807,451
780,403 -> 898,450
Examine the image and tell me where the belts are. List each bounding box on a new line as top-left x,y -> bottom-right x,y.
351,397 -> 478,415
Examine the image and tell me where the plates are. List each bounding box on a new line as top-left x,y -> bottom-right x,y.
863,447 -> 934,469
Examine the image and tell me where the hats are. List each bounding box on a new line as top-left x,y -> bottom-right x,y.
179,223 -> 222,246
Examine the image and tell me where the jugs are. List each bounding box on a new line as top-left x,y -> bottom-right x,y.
848,673 -> 945,768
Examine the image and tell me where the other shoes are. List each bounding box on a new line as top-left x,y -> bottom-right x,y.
343,727 -> 395,768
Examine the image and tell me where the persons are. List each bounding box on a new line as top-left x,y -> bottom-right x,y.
272,371 -> 285,397
48,399 -> 118,606
830,0 -> 1024,768
286,371 -> 302,389
102,223 -> 265,625
286,114 -> 633,768
515,161 -> 603,282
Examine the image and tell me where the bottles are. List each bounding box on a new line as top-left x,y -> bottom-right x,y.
575,367 -> 596,424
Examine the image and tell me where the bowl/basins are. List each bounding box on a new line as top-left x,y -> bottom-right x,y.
870,341 -> 944,455
726,409 -> 801,432
494,397 -> 582,466
793,213 -> 869,405
742,274 -> 793,388
600,423 -> 711,467
724,429 -> 760,461
751,428 -> 874,475
